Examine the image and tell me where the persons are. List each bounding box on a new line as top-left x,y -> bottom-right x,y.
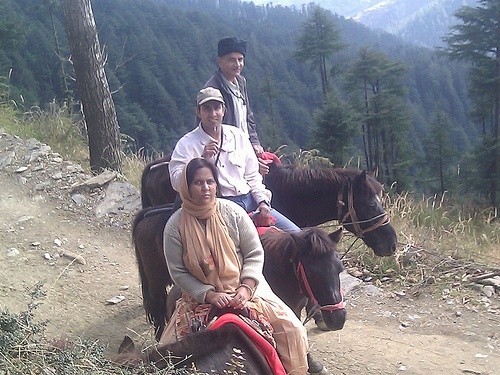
167,86 -> 302,236
155,157 -> 309,375
196,36 -> 273,177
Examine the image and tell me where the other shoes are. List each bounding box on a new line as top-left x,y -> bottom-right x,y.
307,353 -> 322,373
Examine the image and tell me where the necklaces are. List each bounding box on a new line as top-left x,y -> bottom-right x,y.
227,85 -> 248,106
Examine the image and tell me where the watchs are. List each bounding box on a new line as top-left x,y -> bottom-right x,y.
259,200 -> 270,206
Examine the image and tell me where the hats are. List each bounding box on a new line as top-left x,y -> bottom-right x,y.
217,36 -> 247,56
196,87 -> 224,107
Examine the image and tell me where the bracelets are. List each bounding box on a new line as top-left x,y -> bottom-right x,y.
235,284 -> 254,300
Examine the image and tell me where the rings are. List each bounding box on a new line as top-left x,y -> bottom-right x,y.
239,299 -> 243,303
240,303 -> 245,306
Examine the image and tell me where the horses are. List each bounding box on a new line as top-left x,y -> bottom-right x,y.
106,312 -> 288,375
132,203 -> 347,375
139,157 -> 397,257
